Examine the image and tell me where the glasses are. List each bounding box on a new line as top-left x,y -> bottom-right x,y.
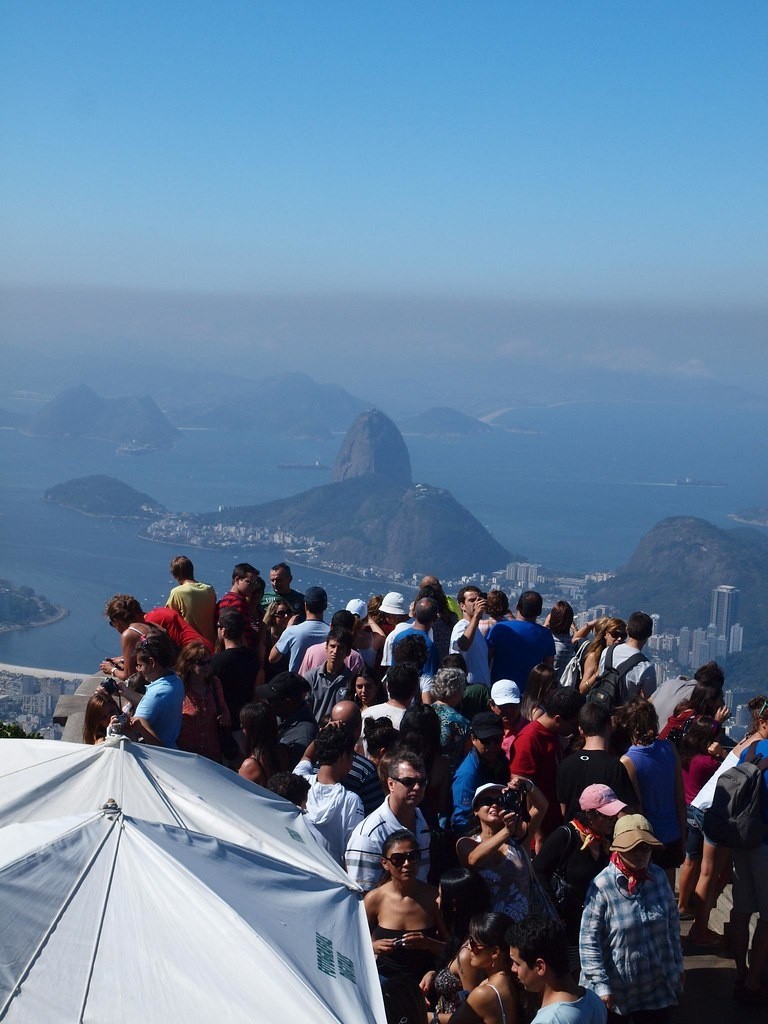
607,630 -> 628,640
389,775 -> 428,788
384,850 -> 421,866
188,659 -> 214,666
240,579 -> 256,587
475,737 -> 503,747
216,624 -> 225,628
275,609 -> 293,617
109,618 -> 119,627
239,722 -> 245,728
758,699 -> 768,718
475,796 -> 504,806
594,811 -> 619,820
468,936 -> 502,956
629,843 -> 652,852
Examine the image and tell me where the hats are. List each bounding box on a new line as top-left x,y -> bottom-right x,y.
255,671 -> 312,704
302,586 -> 410,620
579,783 -> 663,852
471,679 -> 521,806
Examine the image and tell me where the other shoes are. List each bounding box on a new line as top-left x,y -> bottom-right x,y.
678,891 -> 768,1004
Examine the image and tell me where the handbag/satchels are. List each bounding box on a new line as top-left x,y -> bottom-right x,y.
667,711 -> 699,748
514,845 -> 567,948
548,825 -> 571,904
558,640 -> 591,688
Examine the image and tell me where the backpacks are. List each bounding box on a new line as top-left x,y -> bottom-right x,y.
705,740 -> 768,850
585,644 -> 651,710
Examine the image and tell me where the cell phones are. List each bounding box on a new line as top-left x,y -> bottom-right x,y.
479,593 -> 487,599
354,693 -> 358,704
105,657 -> 121,670
392,939 -> 402,946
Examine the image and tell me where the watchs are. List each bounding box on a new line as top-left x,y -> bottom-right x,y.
110,667 -> 118,677
528,778 -> 535,795
430,1011 -> 441,1024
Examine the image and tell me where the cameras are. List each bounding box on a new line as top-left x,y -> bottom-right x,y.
117,713 -> 128,728
101,677 -> 119,695
297,612 -> 306,623
497,788 -> 521,814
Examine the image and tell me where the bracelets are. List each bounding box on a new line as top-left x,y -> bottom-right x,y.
584,680 -> 591,691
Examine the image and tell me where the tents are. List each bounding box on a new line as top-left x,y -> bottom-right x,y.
0,734 -> 388,1024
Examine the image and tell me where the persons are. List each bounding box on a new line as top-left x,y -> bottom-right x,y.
578,813 -> 694,1024
425,911 -> 529,1024
505,914 -> 607,1024
82,554 -> 766,1023
